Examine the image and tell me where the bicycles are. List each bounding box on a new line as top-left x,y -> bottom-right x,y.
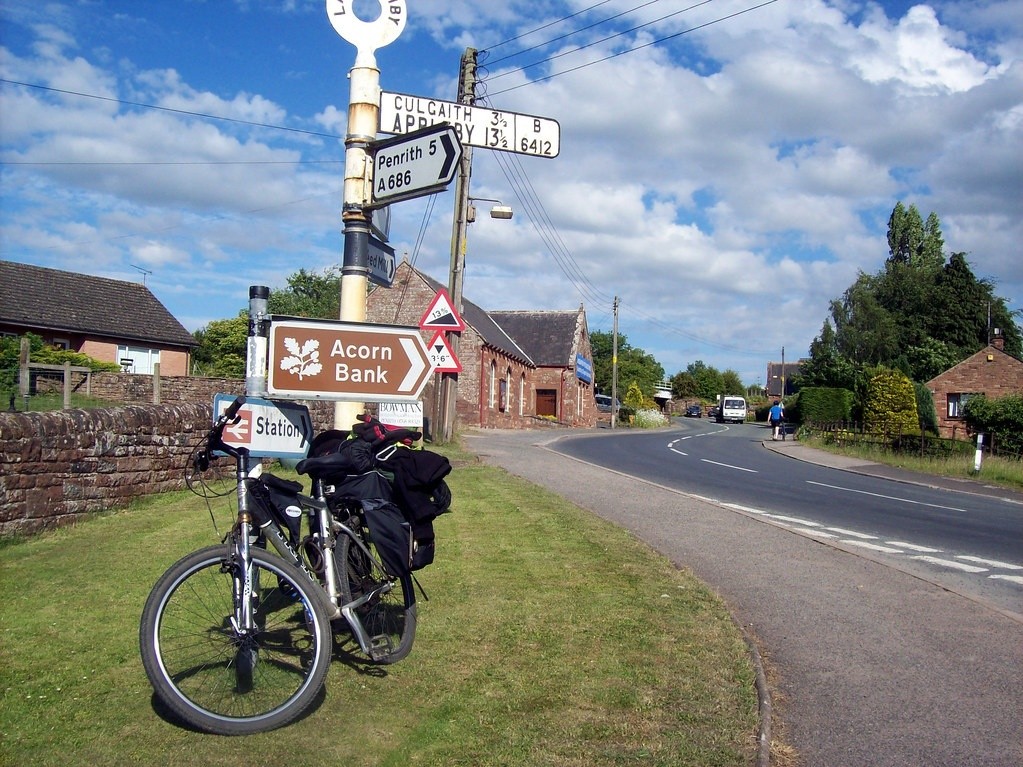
139,394 -> 417,735
781,418 -> 787,440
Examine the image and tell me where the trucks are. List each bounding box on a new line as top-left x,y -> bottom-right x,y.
712,396 -> 746,423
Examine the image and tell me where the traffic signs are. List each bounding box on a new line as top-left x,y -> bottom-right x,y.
268,318 -> 435,399
368,123 -> 465,200
366,238 -> 398,288
208,396 -> 311,459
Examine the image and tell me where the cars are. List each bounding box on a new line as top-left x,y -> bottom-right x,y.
686,406 -> 702,418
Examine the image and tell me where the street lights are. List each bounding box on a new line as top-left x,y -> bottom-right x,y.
773,375 -> 784,400
443,198 -> 513,440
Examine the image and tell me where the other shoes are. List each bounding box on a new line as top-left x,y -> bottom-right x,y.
773,438 -> 778,441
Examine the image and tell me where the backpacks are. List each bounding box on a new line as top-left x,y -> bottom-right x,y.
310,414 -> 451,575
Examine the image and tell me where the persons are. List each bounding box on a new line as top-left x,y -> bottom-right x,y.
768,401 -> 785,440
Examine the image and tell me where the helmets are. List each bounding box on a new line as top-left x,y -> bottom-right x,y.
774,401 -> 779,405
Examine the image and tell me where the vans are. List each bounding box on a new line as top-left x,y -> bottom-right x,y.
595,395 -> 623,413
